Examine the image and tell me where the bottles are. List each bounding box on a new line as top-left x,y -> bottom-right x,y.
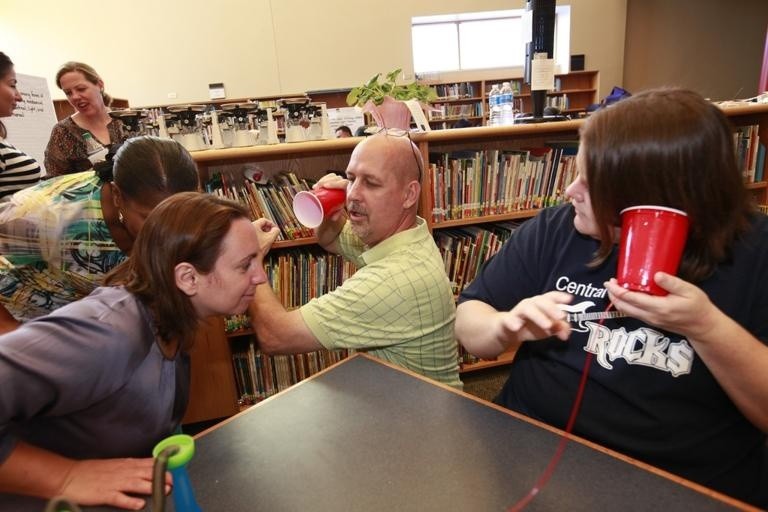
82,131 -> 109,166
500,82 -> 514,126
488,84 -> 502,127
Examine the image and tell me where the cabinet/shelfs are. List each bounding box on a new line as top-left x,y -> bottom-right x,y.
109,69 -> 766,413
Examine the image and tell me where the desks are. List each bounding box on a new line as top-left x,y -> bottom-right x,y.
125,350 -> 763,510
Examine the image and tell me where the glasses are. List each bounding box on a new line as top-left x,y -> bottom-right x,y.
364,126 -> 421,182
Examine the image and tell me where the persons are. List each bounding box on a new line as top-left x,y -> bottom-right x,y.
0,50 -> 42,207
452,85 -> 766,512
42,61 -> 128,178
0,135 -> 201,342
245,126 -> 465,392
0,189 -> 268,512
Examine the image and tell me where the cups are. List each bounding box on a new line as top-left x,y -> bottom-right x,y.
292,188 -> 347,229
616,205 -> 691,297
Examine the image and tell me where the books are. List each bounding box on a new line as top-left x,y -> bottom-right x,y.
427,80 -> 524,119
727,116 -> 765,189
423,140 -> 579,299
544,78 -> 568,114
112,96 -> 365,152
224,246 -> 359,332
232,337 -> 358,409
205,164 -> 348,241
